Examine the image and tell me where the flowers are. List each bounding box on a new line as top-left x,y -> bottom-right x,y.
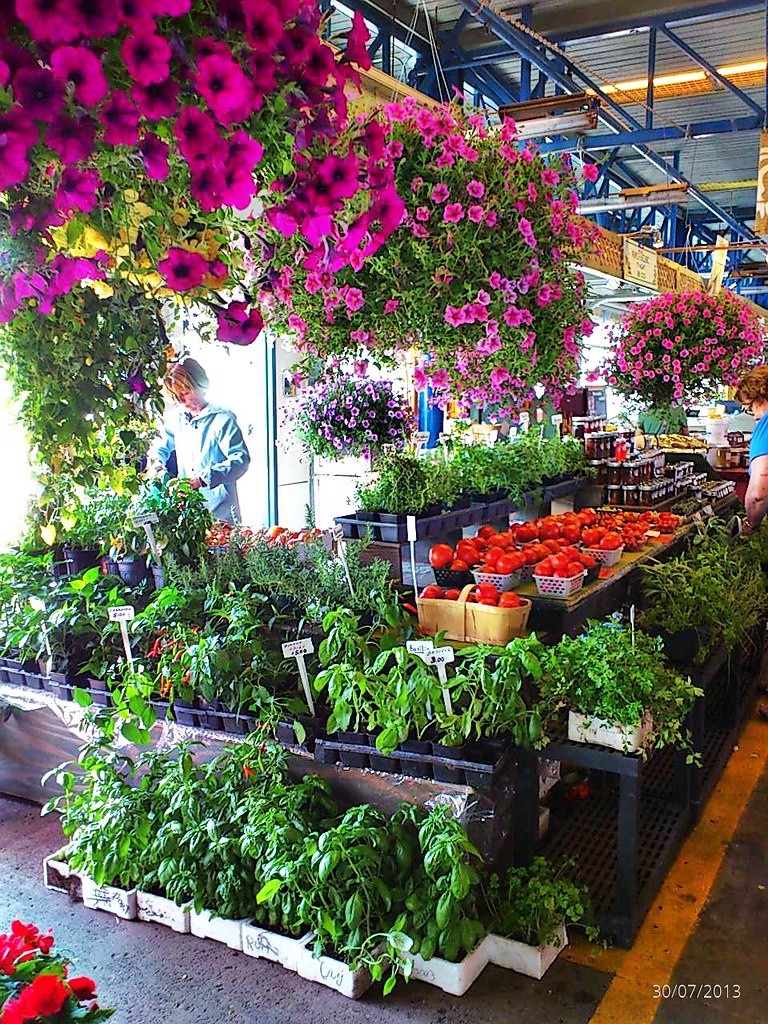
0,3 -> 768,460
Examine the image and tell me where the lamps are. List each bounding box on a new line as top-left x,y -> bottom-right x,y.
585,70 -> 707,96
703,61 -> 767,84
480,109 -> 600,141
570,181 -> 693,214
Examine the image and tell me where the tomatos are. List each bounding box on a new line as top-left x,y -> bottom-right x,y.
419,507 -> 623,608
203,522 -> 326,549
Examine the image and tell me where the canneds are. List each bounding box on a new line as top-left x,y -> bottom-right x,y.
572,415 -> 707,506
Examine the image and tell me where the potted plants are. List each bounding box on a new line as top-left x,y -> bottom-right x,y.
0,479 -> 766,1003
354,417 -> 599,524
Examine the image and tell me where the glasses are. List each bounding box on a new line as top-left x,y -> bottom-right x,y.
740,395 -> 759,411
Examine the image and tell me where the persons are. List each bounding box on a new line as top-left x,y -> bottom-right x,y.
732,365 -> 768,718
637,395 -> 689,436
148,359 -> 250,525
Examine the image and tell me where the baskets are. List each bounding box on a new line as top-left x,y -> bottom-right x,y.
430,537 -> 559,594
531,563 -> 587,597
579,542 -> 624,567
